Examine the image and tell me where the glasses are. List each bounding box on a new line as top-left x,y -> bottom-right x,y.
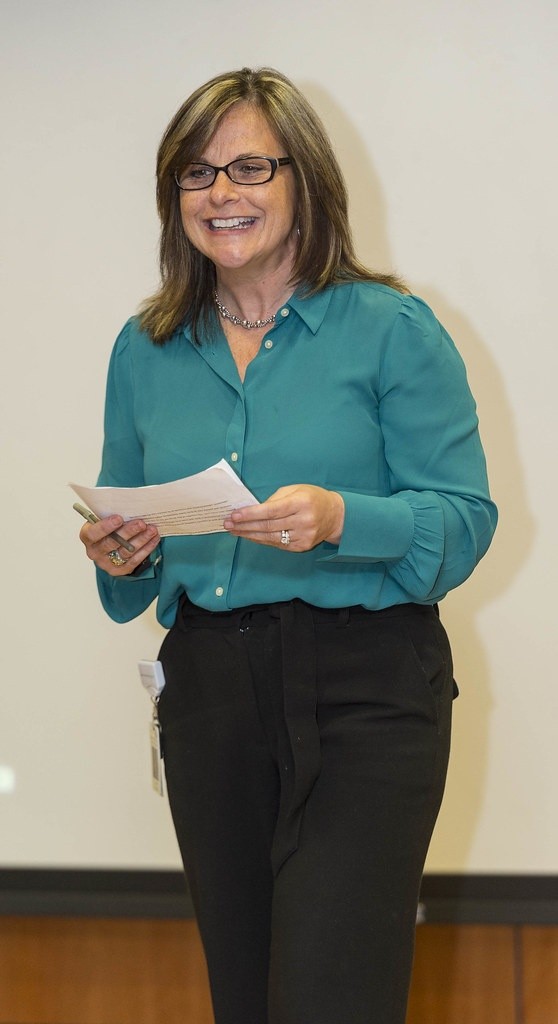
171,155 -> 293,190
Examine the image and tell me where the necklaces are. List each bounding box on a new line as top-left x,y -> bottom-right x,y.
214,292 -> 276,327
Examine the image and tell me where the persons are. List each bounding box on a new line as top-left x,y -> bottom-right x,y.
79,66 -> 499,1023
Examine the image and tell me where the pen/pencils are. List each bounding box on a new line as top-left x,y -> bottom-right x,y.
73,503 -> 136,553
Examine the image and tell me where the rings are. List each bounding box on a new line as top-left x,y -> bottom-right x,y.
281,531 -> 291,545
109,551 -> 126,567
